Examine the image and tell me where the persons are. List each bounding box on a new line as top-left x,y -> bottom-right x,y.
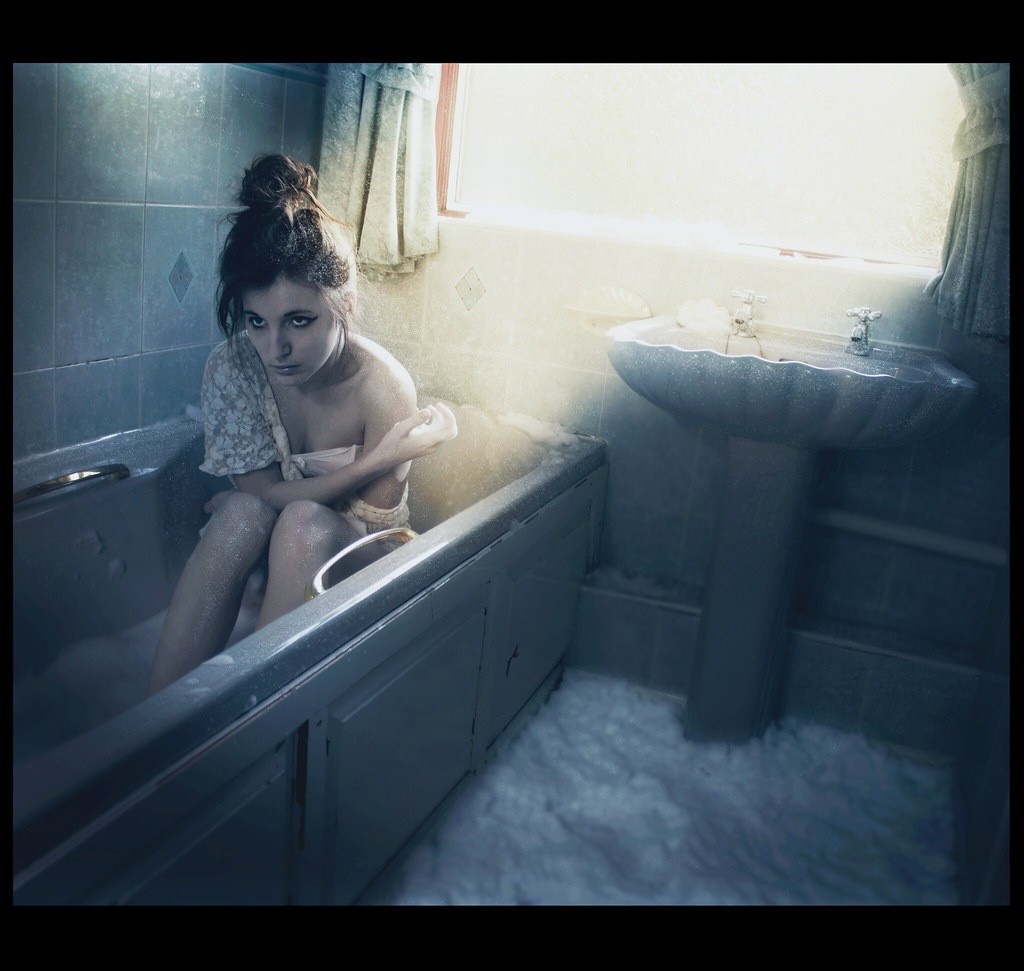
141,152 -> 458,703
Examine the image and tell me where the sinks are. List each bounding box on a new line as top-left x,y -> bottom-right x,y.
599,309 -> 978,452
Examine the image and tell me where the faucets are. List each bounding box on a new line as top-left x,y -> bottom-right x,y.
840,305 -> 881,355
730,290 -> 769,337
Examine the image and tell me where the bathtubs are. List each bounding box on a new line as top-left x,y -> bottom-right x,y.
14,353 -> 612,905
